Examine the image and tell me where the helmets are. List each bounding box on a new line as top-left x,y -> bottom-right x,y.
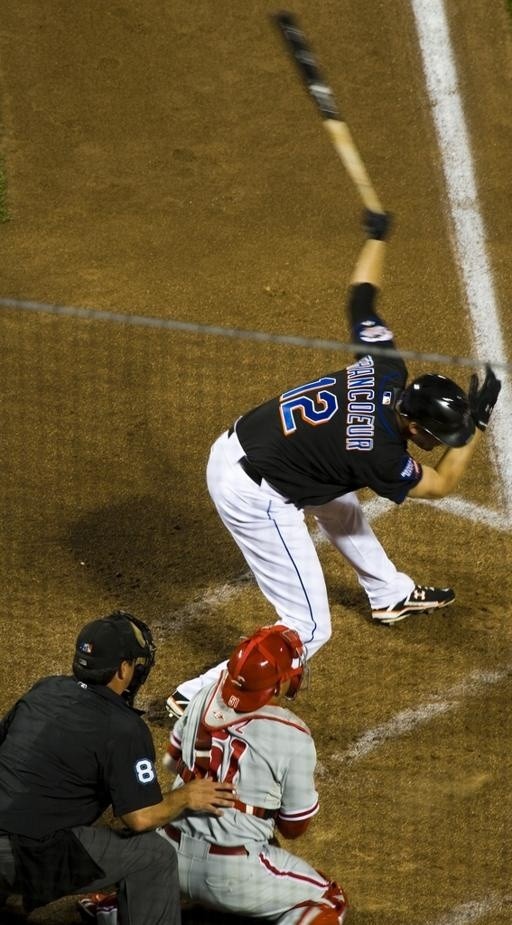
70,611 -> 155,696
396,373 -> 477,448
220,623 -> 307,714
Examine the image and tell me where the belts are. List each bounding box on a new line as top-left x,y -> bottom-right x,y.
162,822 -> 249,856
227,424 -> 293,506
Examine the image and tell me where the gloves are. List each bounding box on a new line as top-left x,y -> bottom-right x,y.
360,207 -> 394,243
468,361 -> 502,433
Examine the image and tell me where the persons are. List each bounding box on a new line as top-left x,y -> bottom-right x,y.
77,626 -> 348,925
0,611 -> 239,925
166,208 -> 502,720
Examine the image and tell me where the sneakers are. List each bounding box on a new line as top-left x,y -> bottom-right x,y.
370,586 -> 456,628
76,893 -> 119,924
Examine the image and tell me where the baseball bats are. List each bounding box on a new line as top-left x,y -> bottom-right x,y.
272,7 -> 383,221
